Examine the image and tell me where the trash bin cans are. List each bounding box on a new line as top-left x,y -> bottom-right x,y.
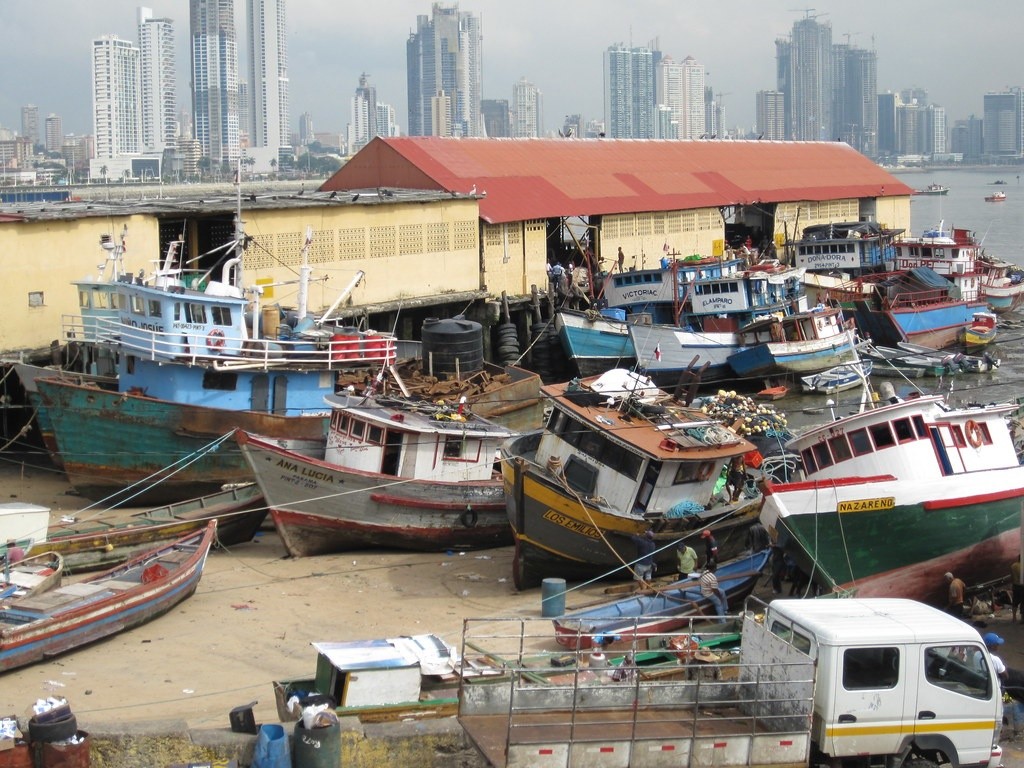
334,333 -> 346,362
345,335 -> 361,359
364,334 -> 380,358
42,731 -> 89,768
250,724 -> 292,768
0,739 -> 33,768
293,720 -> 341,768
379,341 -> 395,365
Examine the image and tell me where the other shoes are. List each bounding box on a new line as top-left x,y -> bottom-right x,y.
725,502 -> 731,506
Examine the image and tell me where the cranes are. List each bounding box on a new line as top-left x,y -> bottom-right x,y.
786,8 -> 816,19
809,13 -> 830,20
841,31 -> 862,45
778,30 -> 792,42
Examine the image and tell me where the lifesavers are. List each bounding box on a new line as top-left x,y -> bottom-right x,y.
496,322 -> 522,366
964,419 -> 983,448
532,322 -> 560,375
460,508 -> 478,527
207,329 -> 225,351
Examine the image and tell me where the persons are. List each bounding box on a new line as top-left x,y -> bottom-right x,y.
725,235 -> 752,261
0,542 -> 24,567
725,454 -> 747,505
599,257 -> 606,272
944,572 -> 966,620
1011,555 -> 1024,624
973,633 -> 1008,698
617,247 -> 624,274
633,530 -> 656,590
701,530 -> 719,571
547,258 -> 576,293
676,543 -> 731,623
836,316 -> 855,333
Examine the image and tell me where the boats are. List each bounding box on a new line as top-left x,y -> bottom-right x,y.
232,304 -> 521,558
553,543 -> 774,652
0,550 -> 65,606
604,632 -> 740,673
913,181 -> 950,195
496,322 -> 768,594
270,632 -> 600,724
757,311 -> 1024,604
23,481 -> 269,577
0,518 -> 219,674
0,535 -> 35,569
799,358 -> 875,396
984,191 -> 1007,202
555,209 -> 1024,392
756,384 -> 787,400
8,157 -> 549,508
957,312 -> 997,346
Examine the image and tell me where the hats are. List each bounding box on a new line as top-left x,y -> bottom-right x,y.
645,531 -> 653,539
677,542 -> 685,550
702,530 -> 710,538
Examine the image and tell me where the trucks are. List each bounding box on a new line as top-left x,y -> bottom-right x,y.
454,592 -> 997,768
